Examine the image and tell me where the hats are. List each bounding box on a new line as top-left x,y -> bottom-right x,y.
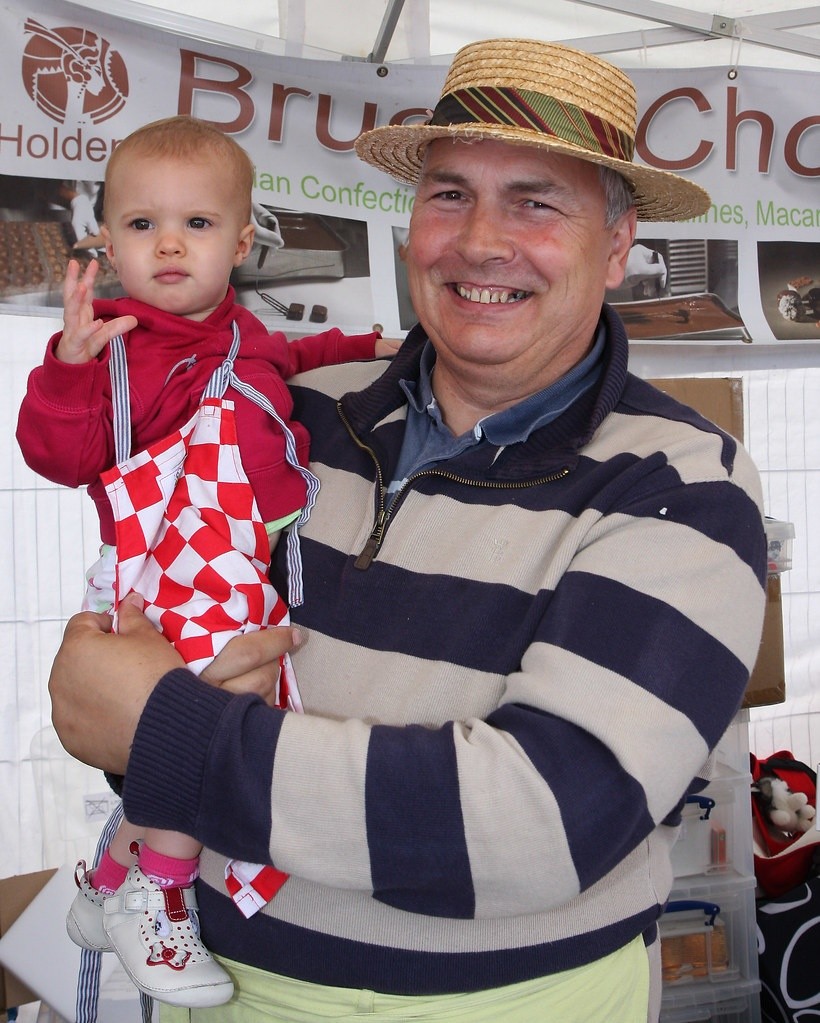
353,37 -> 711,222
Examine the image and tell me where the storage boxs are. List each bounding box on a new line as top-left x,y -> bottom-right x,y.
646,378 -> 786,1023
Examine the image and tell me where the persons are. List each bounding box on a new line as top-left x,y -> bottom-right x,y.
38,179 -> 105,258
621,244 -> 667,296
48,40 -> 768,1023
16,117 -> 407,1007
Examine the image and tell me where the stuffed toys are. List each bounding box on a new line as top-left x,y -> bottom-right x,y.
761,777 -> 815,831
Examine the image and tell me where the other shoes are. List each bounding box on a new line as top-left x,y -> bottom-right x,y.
102,862 -> 235,1007
66,859 -> 116,953
